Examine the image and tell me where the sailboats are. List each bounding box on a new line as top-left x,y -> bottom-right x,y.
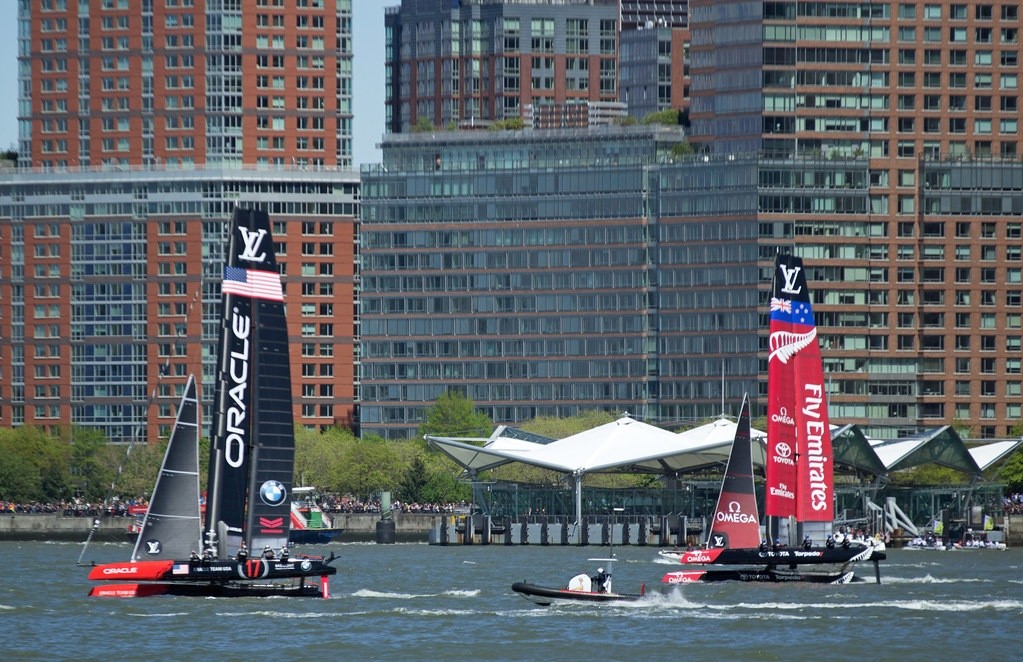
76,201 -> 342,599
658,231 -> 886,586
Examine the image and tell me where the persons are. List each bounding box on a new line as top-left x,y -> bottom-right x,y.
597,568 -> 605,593
0,498 -> 473,519
189,545 -> 291,563
671,493 -> 1023,555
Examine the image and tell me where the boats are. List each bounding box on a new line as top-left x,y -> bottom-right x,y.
511,558 -> 663,607
901,544 -> 980,551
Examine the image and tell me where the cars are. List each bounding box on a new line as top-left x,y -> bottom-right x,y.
104,504 -> 125,517
458,520 -> 505,533
68,504 -> 89,518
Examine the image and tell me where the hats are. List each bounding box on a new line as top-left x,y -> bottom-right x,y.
191,550 -> 195,554
265,545 -> 270,549
806,534 -> 809,537
844,533 -> 847,536
242,541 -> 246,545
775,539 -> 780,543
828,534 -> 831,537
204,551 -> 209,554
283,545 -> 286,548
762,538 -> 766,543
597,568 -> 603,573
